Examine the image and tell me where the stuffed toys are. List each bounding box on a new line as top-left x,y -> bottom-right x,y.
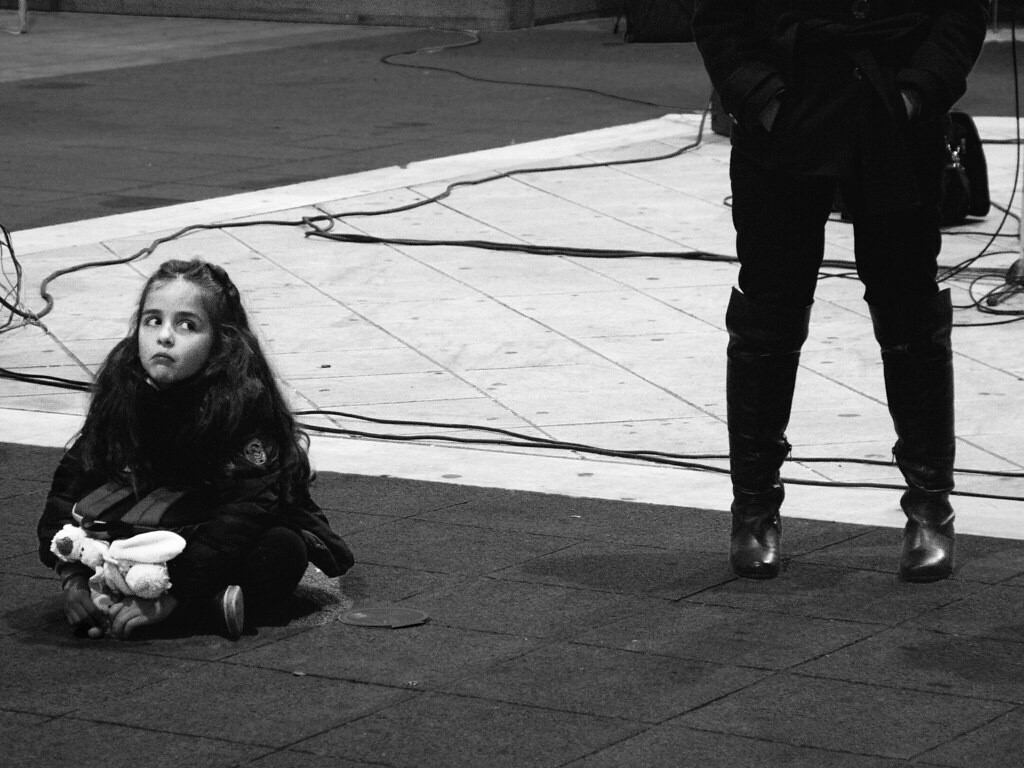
48,522 -> 188,634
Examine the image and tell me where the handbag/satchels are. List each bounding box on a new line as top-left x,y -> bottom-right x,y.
937,112 -> 987,225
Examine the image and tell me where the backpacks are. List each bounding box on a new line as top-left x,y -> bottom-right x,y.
72,480 -> 193,538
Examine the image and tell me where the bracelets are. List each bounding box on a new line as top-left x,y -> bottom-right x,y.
61,571 -> 91,590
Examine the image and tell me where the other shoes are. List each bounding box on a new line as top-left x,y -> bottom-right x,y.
184,585 -> 245,635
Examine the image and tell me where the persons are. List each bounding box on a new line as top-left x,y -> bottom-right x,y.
35,258 -> 354,637
690,1 -> 990,579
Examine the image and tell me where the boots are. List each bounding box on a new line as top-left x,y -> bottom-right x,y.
726,286 -> 814,575
864,289 -> 955,581
